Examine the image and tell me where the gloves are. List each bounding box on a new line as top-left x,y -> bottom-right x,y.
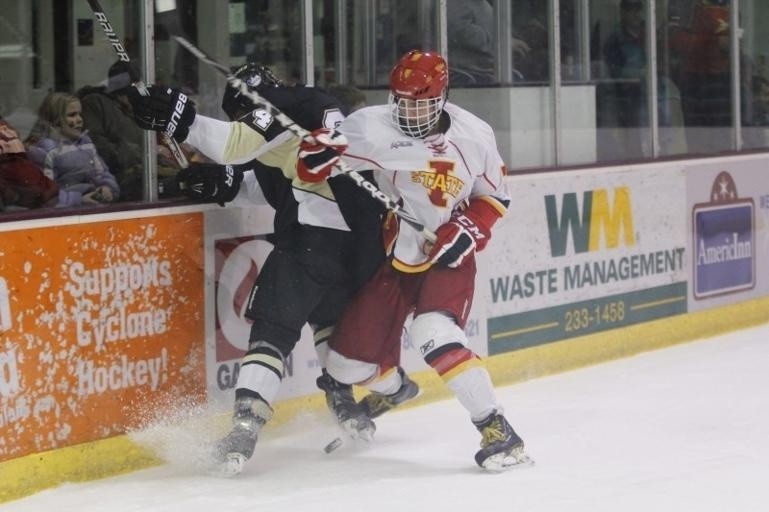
297,128 -> 349,183
176,162 -> 244,202
127,83 -> 197,143
423,194 -> 507,270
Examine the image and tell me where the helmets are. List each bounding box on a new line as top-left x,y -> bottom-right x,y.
390,49 -> 450,99
222,62 -> 284,121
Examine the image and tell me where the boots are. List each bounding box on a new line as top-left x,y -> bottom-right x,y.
316,366 -> 419,437
471,408 -> 524,468
215,388 -> 274,460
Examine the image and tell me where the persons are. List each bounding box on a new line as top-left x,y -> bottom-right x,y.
296,47 -> 525,468
127,59 -> 387,478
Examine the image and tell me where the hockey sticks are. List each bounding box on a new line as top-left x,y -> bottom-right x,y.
155,0 -> 438,245
